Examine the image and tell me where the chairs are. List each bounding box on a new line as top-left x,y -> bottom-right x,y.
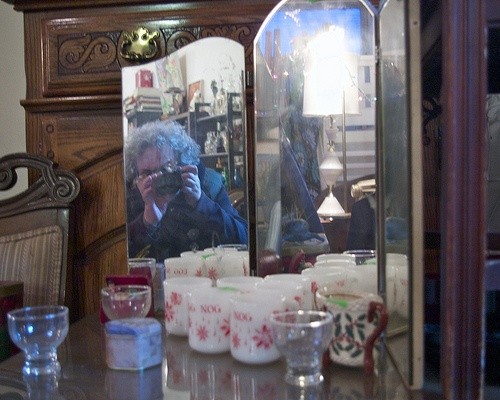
0,153 -> 84,306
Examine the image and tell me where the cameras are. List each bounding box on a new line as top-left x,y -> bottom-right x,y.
155,166 -> 188,193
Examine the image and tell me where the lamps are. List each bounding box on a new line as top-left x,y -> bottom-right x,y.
316,115 -> 349,217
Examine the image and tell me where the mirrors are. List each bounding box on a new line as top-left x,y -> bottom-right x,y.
121,1 -> 424,387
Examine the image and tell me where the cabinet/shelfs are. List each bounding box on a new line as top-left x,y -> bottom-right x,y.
161,92 -> 244,192
5,1 -> 305,319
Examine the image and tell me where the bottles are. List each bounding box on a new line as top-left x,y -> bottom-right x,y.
221,163 -> 229,191
105,317 -> 161,370
215,121 -> 224,153
233,168 -> 241,188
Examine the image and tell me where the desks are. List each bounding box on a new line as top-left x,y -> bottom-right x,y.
0,301 -> 423,400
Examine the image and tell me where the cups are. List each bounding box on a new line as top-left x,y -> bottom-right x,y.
129,246 -> 249,280
101,285 -> 152,319
163,272 -> 384,387
8,305 -> 70,376
302,249 -> 409,320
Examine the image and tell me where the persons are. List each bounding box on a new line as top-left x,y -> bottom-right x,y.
124,122 -> 248,259
